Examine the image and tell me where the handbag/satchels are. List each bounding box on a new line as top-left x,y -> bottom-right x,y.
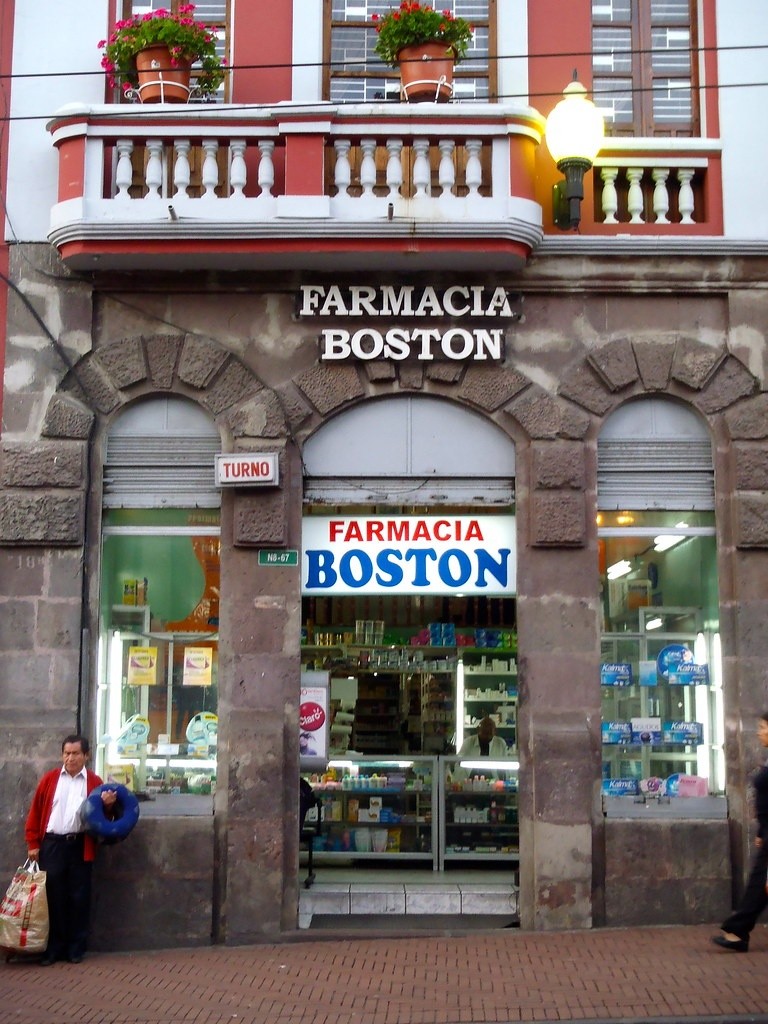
0,857 -> 49,953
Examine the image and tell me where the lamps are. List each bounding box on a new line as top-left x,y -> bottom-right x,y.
546,69 -> 605,231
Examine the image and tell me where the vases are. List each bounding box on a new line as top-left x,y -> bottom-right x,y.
134,44 -> 191,103
396,40 -> 459,103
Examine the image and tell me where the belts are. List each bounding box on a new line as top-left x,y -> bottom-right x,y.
44,833 -> 83,841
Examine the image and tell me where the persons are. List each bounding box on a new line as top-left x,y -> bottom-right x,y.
709,711 -> 768,949
456,718 -> 509,759
24,735 -> 117,965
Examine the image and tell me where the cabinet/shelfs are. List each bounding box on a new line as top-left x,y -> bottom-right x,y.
114,756 -> 438,870
301,645 -> 457,755
457,646 -> 518,749
439,755 -> 519,871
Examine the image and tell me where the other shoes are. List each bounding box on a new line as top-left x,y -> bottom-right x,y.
42,953 -> 56,965
711,935 -> 749,953
71,953 -> 80,963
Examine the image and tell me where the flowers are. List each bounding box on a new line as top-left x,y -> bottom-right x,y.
96,3 -> 229,97
372,0 -> 474,69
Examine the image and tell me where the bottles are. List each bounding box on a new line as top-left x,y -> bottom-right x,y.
192,714 -> 210,757
301,766 -> 391,790
450,798 -> 518,825
443,773 -> 519,794
319,798 -> 333,823
